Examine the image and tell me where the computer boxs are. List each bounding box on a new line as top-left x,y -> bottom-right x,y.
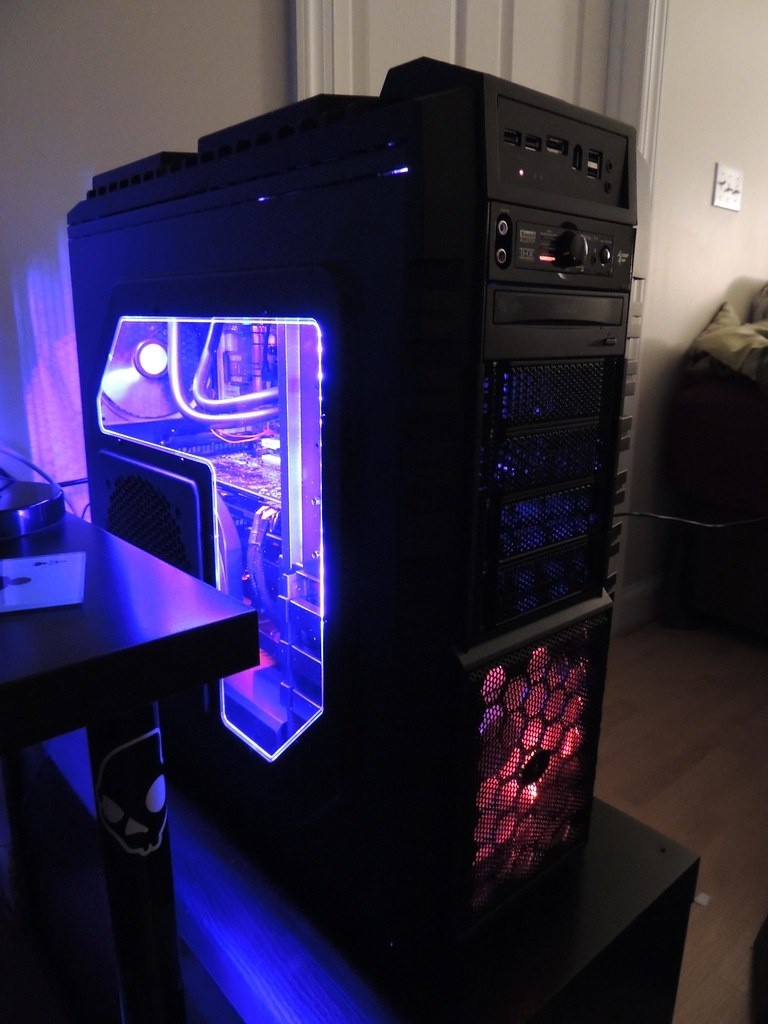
65,57 -> 638,991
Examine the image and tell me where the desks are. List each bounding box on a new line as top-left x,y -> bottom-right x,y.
0,514 -> 260,1024
385,797 -> 700,1024
